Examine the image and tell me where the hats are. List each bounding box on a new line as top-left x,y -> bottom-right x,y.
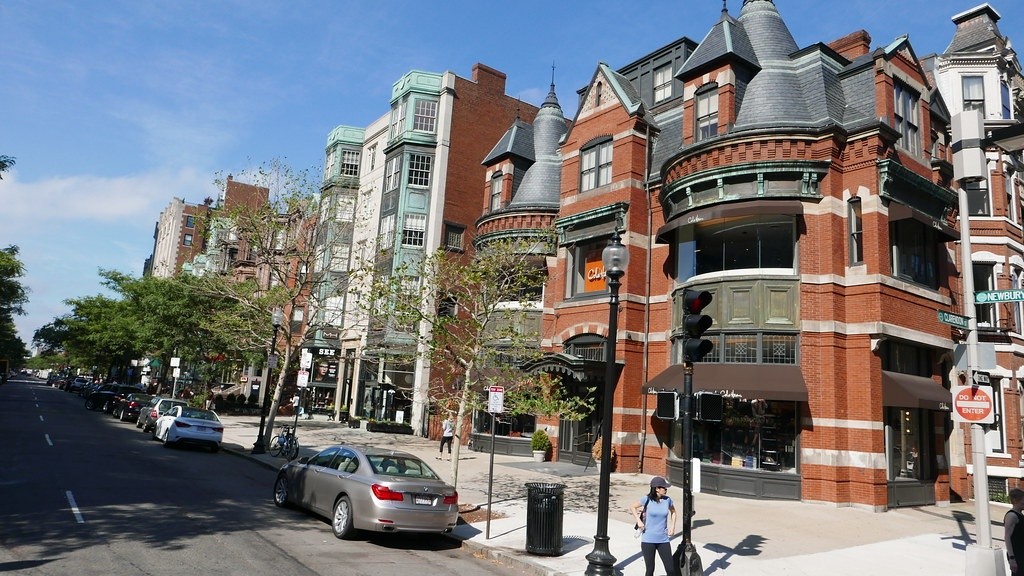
650,477 -> 671,488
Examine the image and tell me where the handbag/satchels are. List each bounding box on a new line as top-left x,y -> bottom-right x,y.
635,512 -> 645,529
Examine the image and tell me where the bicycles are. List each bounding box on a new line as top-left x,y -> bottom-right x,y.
268,422 -> 303,463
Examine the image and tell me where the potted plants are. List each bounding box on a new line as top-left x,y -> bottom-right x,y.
530,429 -> 551,462
327,402 -> 335,419
592,435 -> 615,473
340,404 -> 349,422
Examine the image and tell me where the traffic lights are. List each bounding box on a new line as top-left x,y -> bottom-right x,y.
680,287 -> 716,367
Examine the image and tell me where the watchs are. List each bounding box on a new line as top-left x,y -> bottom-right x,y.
1009,555 -> 1015,560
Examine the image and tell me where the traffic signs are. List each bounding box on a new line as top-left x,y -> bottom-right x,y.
972,370 -> 993,389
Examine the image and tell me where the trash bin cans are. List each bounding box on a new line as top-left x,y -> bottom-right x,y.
524,483 -> 568,556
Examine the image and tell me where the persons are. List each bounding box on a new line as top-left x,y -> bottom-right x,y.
435,412 -> 454,461
289,393 -> 300,418
1003,488 -> 1024,576
630,477 -> 677,576
308,360 -> 336,383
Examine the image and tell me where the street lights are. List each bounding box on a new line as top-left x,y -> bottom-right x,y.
249,308 -> 285,457
577,212 -> 624,576
948,103 -> 1017,572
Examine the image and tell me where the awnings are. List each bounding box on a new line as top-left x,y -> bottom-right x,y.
881,369 -> 954,412
641,363 -> 809,402
887,202 -> 961,242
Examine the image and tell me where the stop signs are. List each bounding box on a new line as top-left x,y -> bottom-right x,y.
952,386 -> 1002,426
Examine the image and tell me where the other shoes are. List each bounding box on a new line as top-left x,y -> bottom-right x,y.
436,457 -> 442,460
447,459 -> 451,461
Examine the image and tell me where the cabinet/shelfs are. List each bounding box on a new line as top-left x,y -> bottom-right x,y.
758,414 -> 780,470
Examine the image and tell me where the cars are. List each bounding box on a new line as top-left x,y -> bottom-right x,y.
0,359 -> 90,397
85,384 -> 144,415
152,405 -> 225,453
112,392 -> 158,422
273,443 -> 461,539
138,399 -> 190,434
